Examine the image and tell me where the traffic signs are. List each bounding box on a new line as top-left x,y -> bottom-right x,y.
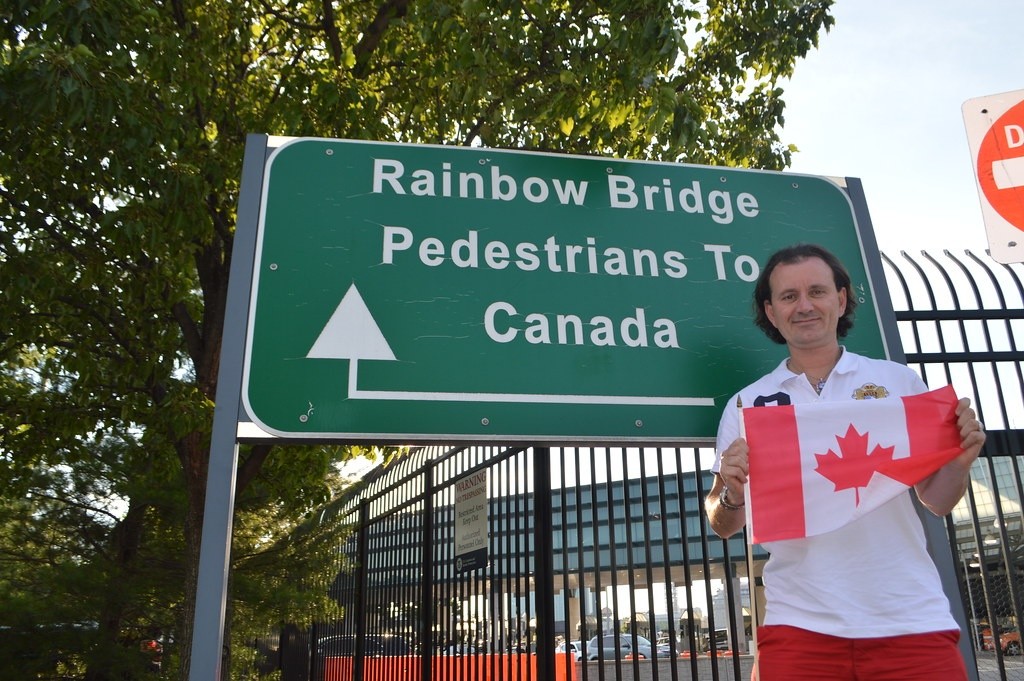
240,137 -> 892,444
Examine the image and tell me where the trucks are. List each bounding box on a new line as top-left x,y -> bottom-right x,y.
714,629 -> 729,651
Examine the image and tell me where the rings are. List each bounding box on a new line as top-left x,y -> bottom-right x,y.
977,420 -> 983,431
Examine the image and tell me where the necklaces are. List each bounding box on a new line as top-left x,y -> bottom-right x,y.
787,358 -> 839,393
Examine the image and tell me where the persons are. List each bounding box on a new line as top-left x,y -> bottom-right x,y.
704,242 -> 986,681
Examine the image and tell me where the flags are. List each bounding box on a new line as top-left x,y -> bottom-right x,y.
743,385 -> 969,545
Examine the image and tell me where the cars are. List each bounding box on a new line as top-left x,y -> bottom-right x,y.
656,637 -> 670,653
317,632 -> 536,656
997,618 -> 1023,657
554,641 -> 588,663
586,634 -> 680,659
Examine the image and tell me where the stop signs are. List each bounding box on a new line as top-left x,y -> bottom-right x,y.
963,85 -> 1024,263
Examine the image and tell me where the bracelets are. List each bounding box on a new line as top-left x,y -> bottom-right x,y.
719,487 -> 744,511
